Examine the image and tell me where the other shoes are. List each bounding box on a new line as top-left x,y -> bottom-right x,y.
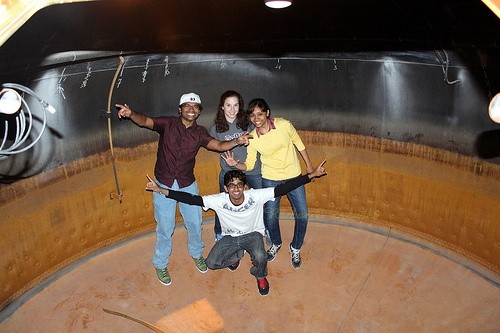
264,229 -> 272,245
215,234 -> 223,241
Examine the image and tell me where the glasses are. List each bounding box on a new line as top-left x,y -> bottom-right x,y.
227,183 -> 244,188
183,104 -> 199,109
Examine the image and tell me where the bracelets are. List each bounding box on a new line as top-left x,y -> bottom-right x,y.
160,187 -> 162,194
234,138 -> 239,145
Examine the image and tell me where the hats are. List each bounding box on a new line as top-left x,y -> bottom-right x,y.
179,92 -> 201,106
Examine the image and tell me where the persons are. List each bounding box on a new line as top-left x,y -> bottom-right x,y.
145,157 -> 328,297
114,91 -> 255,286
219,97 -> 316,271
208,90 -> 261,241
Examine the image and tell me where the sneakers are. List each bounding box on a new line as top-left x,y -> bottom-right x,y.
192,255 -> 208,273
266,242 -> 283,262
289,243 -> 302,269
155,267 -> 172,286
227,260 -> 241,273
258,277 -> 270,297
